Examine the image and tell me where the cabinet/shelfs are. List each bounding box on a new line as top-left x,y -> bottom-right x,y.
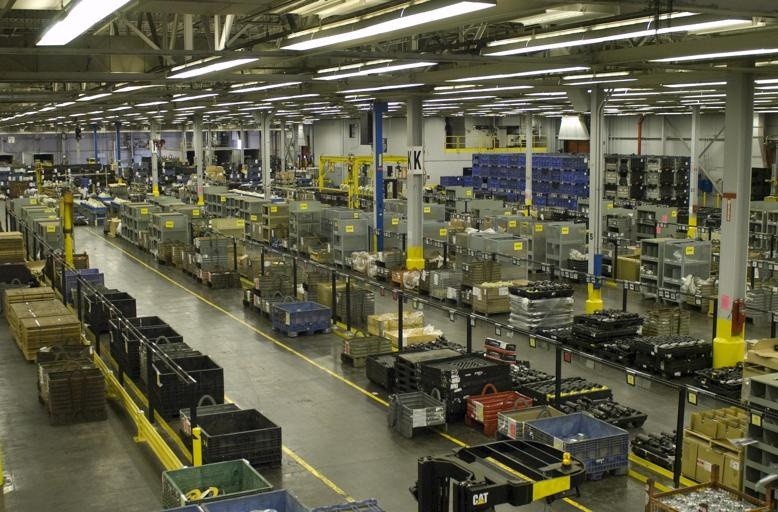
604,153 -> 690,223
741,372 -> 778,508
